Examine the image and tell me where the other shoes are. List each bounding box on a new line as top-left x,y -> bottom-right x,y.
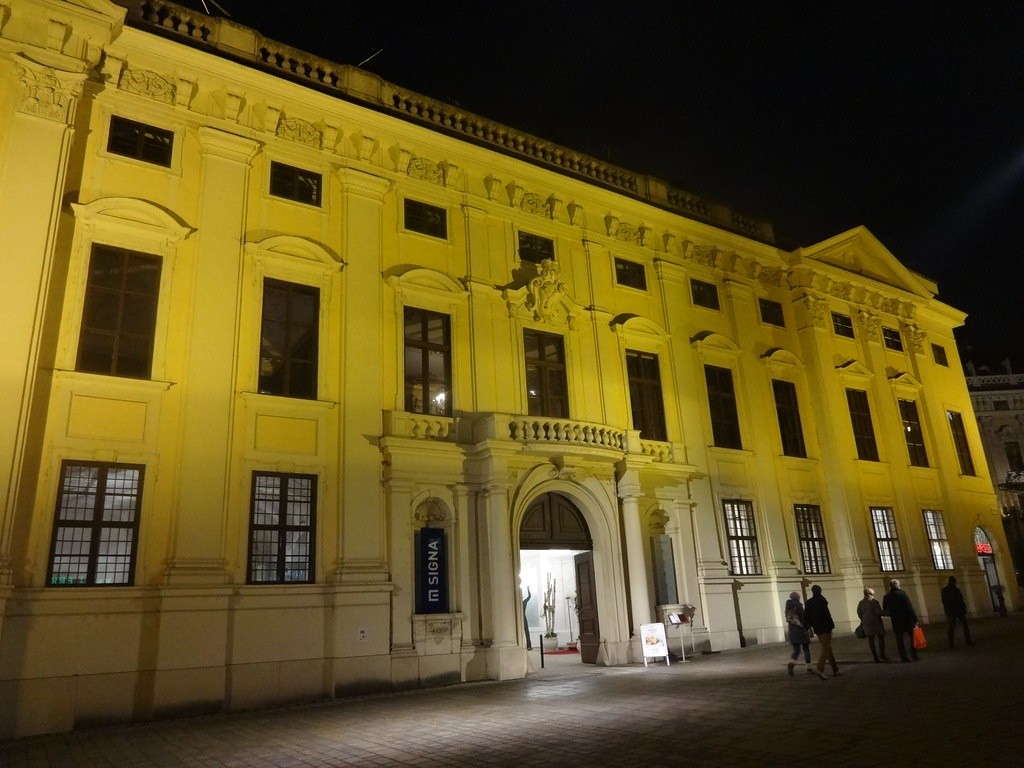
912,654 -> 921,660
832,668 -> 839,676
879,653 -> 889,660
815,670 -> 828,680
949,641 -> 955,648
902,657 -> 912,662
966,640 -> 975,646
874,656 -> 879,663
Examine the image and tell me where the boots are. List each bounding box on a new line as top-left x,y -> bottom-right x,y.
788,658 -> 796,676
805,662 -> 813,674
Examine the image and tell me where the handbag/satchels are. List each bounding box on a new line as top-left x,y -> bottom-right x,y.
787,605 -> 804,628
856,622 -> 865,638
913,623 -> 927,649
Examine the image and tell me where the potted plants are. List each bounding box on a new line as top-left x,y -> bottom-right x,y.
565,596 -> 577,650
574,589 -> 581,653
541,572 -> 558,652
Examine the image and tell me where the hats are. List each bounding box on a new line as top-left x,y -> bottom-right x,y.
790,592 -> 800,600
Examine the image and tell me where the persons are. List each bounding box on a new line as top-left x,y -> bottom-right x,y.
522,586 -> 532,651
855,587 -> 889,664
883,579 -> 921,662
941,576 -> 975,648
784,591 -> 814,675
805,585 -> 843,679
